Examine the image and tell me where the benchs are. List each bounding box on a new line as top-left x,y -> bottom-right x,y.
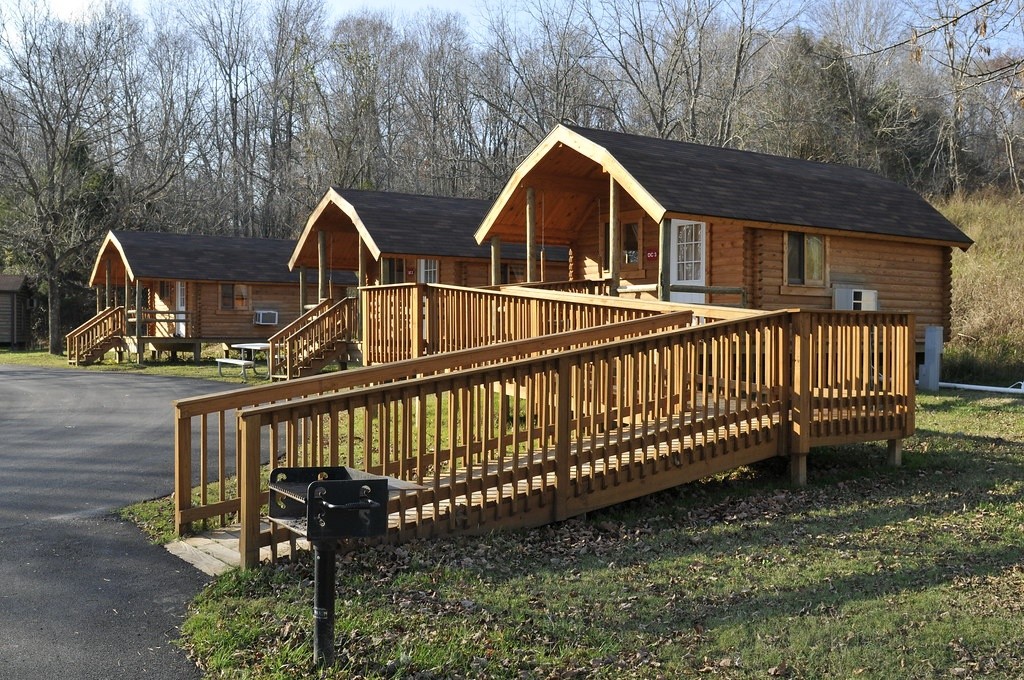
270,355 -> 301,372
215,358 -> 255,382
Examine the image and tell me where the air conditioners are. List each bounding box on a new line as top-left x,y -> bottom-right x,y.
253,310 -> 279,326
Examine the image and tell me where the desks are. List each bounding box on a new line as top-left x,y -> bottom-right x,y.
231,343 -> 288,381
